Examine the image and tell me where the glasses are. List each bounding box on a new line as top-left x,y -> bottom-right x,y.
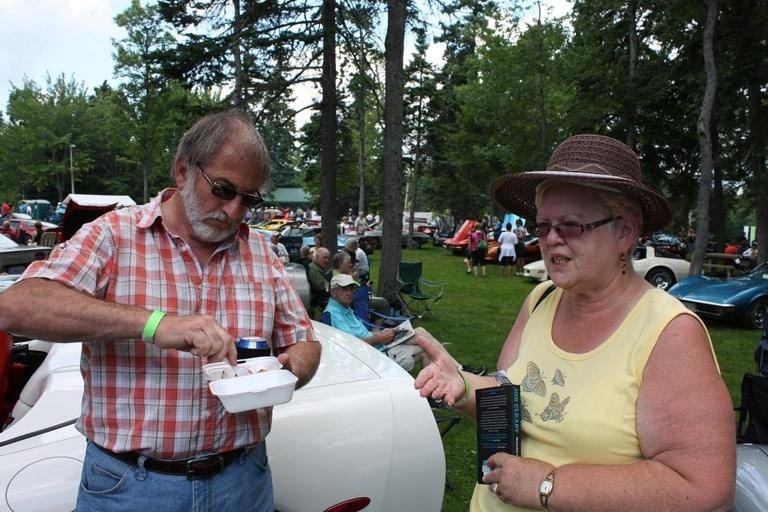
196,161 -> 264,211
530,216 -> 621,238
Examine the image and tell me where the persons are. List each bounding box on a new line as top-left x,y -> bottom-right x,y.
0,200 -> 44,245
0,108 -> 321,512
413,135 -> 737,512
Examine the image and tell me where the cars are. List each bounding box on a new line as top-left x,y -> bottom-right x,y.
278,261 -> 313,315
726,439 -> 768,512
0,207 -> 62,277
650,231 -> 687,254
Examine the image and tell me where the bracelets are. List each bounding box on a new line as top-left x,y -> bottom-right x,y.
142,309 -> 166,344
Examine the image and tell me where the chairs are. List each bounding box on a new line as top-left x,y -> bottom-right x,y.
350,285 -> 414,332
397,261 -> 448,320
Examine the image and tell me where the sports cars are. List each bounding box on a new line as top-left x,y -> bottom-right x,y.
522,242 -> 707,296
0,280 -> 450,511
665,258 -> 767,331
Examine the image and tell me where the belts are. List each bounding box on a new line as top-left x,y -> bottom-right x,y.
92,435 -> 265,481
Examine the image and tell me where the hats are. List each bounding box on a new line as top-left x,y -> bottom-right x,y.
487,134 -> 674,236
330,274 -> 360,288
270,231 -> 281,237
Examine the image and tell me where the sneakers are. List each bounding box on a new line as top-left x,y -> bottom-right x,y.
467,268 -> 525,280
463,364 -> 487,376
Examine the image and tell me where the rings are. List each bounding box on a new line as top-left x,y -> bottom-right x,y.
491,482 -> 500,495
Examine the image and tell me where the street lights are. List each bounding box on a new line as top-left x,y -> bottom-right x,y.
69,143 -> 77,194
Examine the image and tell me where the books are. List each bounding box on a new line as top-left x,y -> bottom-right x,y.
475,385 -> 521,484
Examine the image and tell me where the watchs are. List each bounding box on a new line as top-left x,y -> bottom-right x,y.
538,468 -> 558,512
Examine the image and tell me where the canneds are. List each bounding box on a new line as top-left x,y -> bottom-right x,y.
235,336 -> 271,360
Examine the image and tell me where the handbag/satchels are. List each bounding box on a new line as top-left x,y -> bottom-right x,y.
478,239 -> 488,250
